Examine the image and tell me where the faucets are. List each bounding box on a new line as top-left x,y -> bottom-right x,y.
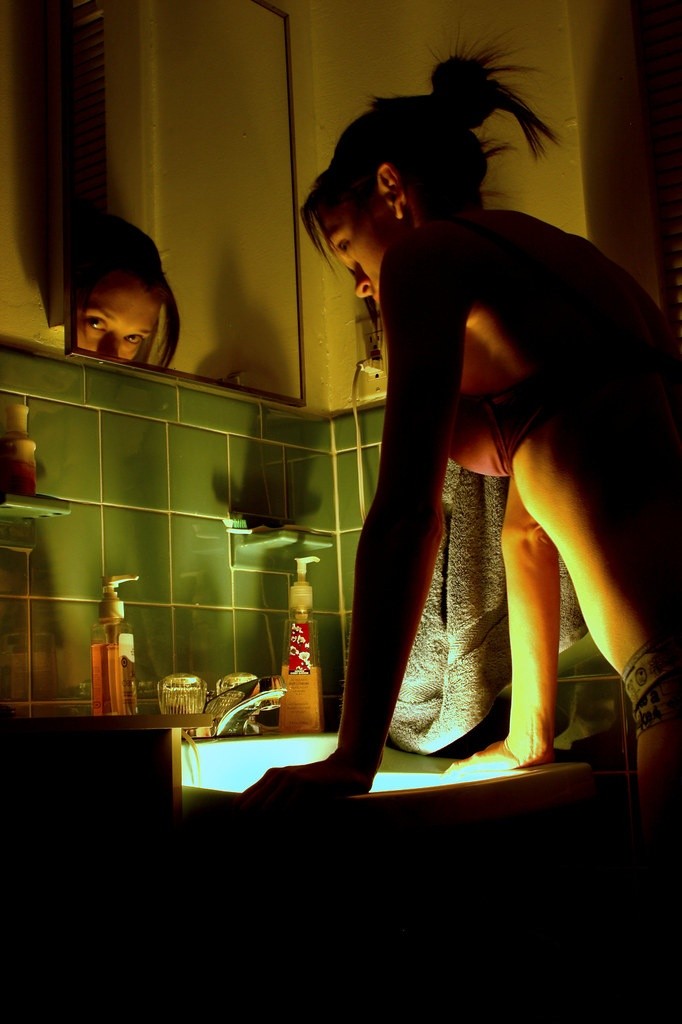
193,674 -> 288,742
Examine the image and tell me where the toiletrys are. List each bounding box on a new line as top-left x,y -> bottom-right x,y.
0,402 -> 39,497
277,554 -> 323,736
86,570 -> 146,718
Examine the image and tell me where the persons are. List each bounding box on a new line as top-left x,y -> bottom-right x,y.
70,214 -> 181,369
239,28 -> 682,847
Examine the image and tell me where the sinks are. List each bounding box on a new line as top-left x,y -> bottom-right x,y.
179,730 -> 529,800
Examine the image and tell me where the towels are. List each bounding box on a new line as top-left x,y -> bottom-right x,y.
386,454 -> 590,758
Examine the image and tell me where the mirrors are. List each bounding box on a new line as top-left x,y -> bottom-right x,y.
52,0 -> 310,412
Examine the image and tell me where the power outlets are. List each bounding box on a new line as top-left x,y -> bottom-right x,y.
356,320 -> 389,403
